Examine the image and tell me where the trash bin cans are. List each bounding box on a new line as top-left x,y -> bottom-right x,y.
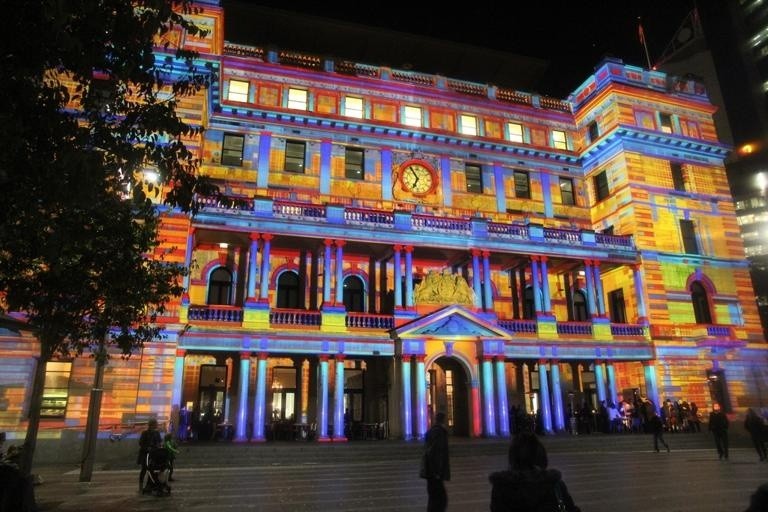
378,430 -> 383,440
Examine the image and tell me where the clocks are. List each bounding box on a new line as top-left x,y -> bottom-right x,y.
397,158 -> 440,199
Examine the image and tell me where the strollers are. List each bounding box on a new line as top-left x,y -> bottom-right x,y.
142,448 -> 171,498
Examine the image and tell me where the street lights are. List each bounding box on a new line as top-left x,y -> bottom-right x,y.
78,166 -> 159,482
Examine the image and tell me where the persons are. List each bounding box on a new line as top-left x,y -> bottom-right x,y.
137,418 -> 179,492
1,446 -> 35,512
568,398 -> 701,434
745,408 -> 766,460
511,404 -> 546,436
488,433 -> 582,512
344,408 -> 353,438
198,408 -> 224,441
648,407 -> 670,453
420,413 -> 449,512
706,406 -> 728,458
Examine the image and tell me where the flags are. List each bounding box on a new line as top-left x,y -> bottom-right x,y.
639,25 -> 643,46
